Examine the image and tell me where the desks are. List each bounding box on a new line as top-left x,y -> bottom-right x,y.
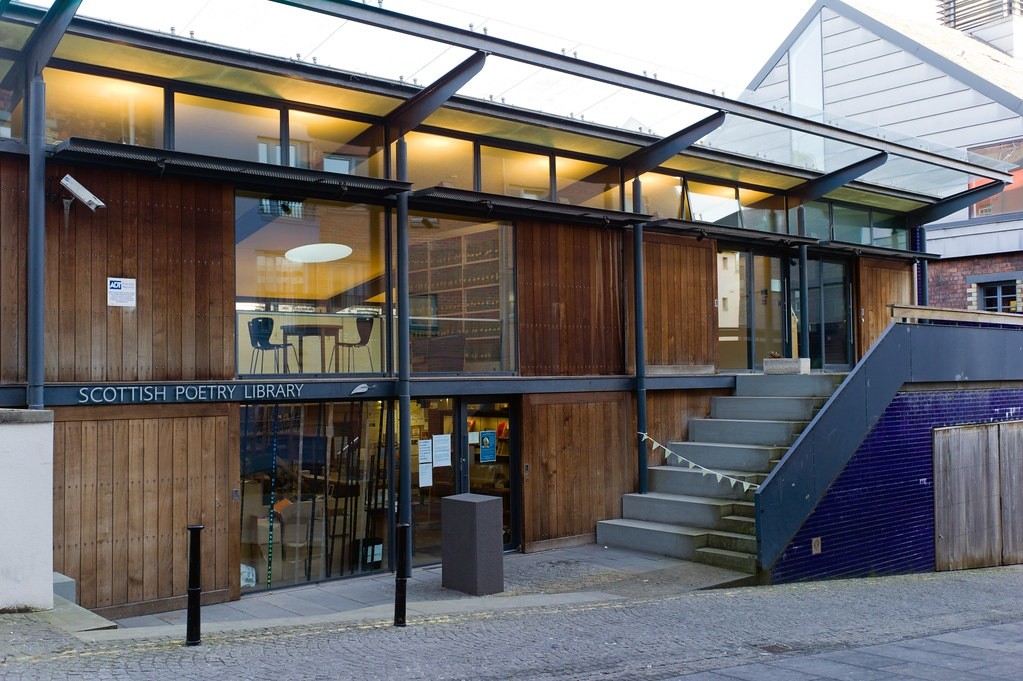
279,325 -> 343,373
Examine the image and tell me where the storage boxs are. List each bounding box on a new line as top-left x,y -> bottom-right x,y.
250,499 -> 352,584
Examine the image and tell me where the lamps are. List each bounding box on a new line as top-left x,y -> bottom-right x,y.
696,228 -> 707,241
599,216 -> 610,231
281,201 -> 291,215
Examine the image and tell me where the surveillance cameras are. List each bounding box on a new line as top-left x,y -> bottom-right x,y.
59,174 -> 107,213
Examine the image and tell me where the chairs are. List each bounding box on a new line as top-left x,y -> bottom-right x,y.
248,317 -> 302,374
329,317 -> 374,373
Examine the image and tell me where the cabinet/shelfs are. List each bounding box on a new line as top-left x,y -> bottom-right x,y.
427,409 -> 510,489
408,222 -> 515,372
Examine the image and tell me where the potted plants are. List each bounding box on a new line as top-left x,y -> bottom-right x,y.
763,351 -> 811,375
